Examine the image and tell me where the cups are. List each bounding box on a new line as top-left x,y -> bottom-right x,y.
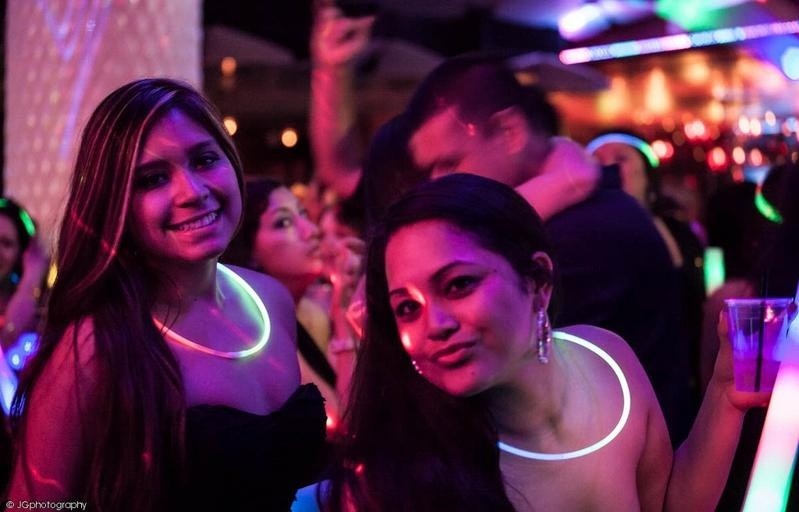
723,298 -> 793,394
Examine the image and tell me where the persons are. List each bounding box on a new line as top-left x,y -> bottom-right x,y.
2,15 -> 799,510
